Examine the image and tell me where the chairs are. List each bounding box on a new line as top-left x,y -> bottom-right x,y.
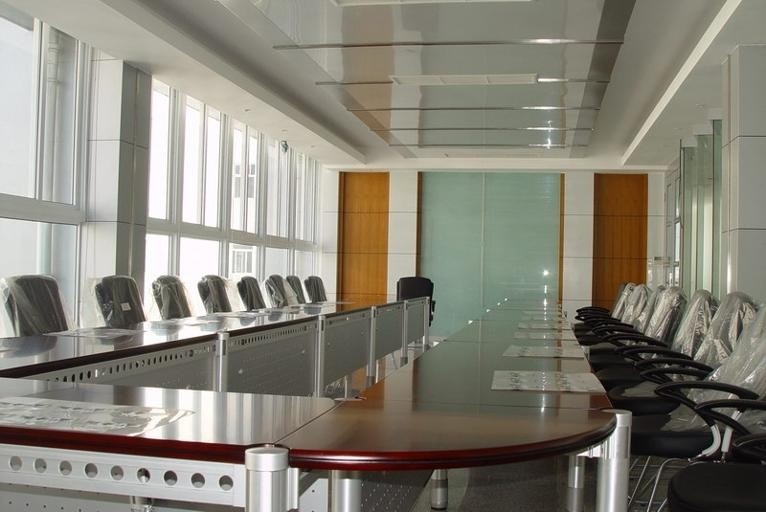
629,310 -> 764,507
647,399 -> 765,512
264,275 -> 288,307
151,275 -> 191,319
576,285 -> 649,339
396,276 -> 435,327
304,276 -> 327,303
197,275 -> 231,314
576,285 -> 666,348
585,286 -> 688,369
236,276 -> 265,311
95,276 -> 145,328
3,275 -> 68,338
574,283 -> 638,331
609,293 -> 755,415
286,276 -> 305,304
593,290 -> 718,386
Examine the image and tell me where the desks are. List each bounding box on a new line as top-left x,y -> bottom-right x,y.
0,295 -> 631,512
